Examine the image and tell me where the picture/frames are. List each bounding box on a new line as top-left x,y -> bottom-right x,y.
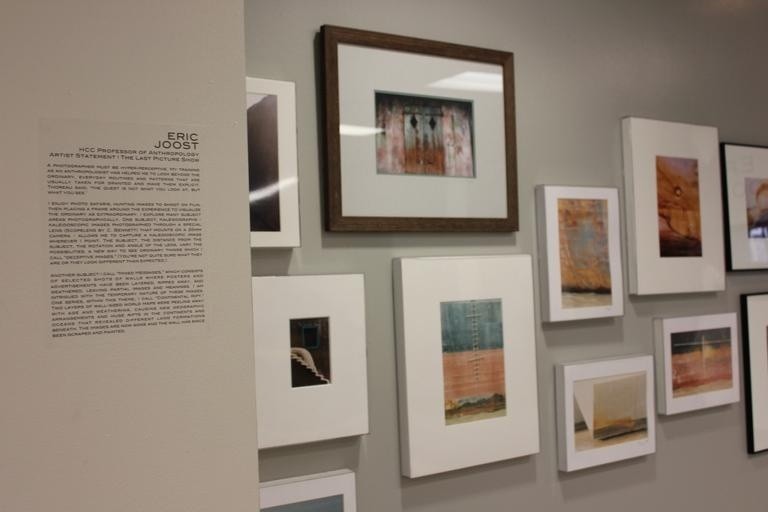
739,290 -> 768,458
720,142 -> 768,273
314,21 -> 519,233
